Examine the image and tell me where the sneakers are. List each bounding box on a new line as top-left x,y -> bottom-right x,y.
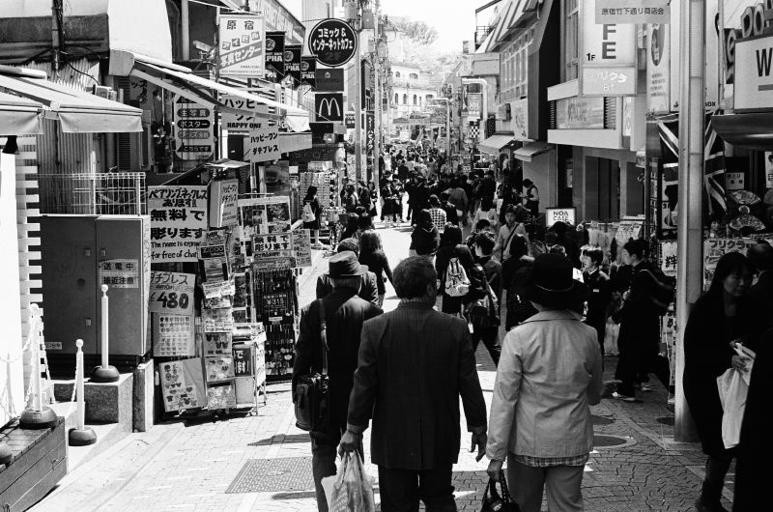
611,391 -> 636,403
637,384 -> 651,391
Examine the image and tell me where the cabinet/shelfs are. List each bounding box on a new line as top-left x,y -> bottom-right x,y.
200,322 -> 268,415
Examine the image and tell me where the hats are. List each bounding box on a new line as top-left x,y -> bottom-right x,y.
512,253 -> 591,308
324,251 -> 368,277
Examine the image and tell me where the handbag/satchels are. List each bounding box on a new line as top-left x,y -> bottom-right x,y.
481,470 -> 520,512
330,481 -> 368,512
293,373 -> 330,430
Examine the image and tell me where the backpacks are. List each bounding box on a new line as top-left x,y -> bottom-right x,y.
641,267 -> 676,317
464,264 -> 501,327
444,257 -> 471,298
300,200 -> 316,223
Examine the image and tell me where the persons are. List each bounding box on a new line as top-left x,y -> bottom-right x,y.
333,256 -> 488,511
728,240 -> 770,512
477,251 -> 606,510
679,249 -> 757,511
289,249 -> 386,512
301,130 -> 677,406
